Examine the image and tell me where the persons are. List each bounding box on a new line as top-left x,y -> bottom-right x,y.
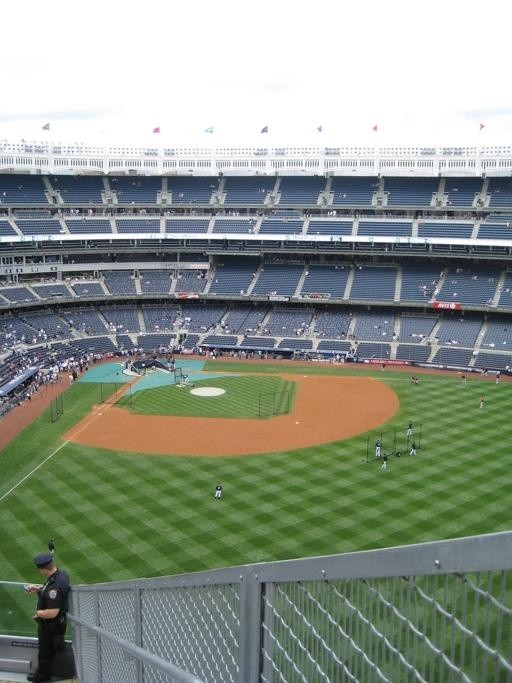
374,440 -> 381,457
214,482 -> 222,499
24,553 -> 70,683
381,453 -> 387,470
409,441 -> 417,455
48,538 -> 55,560
406,421 -> 413,437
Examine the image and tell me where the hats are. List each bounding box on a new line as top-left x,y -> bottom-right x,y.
33,553 -> 54,567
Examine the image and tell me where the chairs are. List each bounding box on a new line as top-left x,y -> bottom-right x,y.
0,170 -> 66,410
66,175 -> 511,356
